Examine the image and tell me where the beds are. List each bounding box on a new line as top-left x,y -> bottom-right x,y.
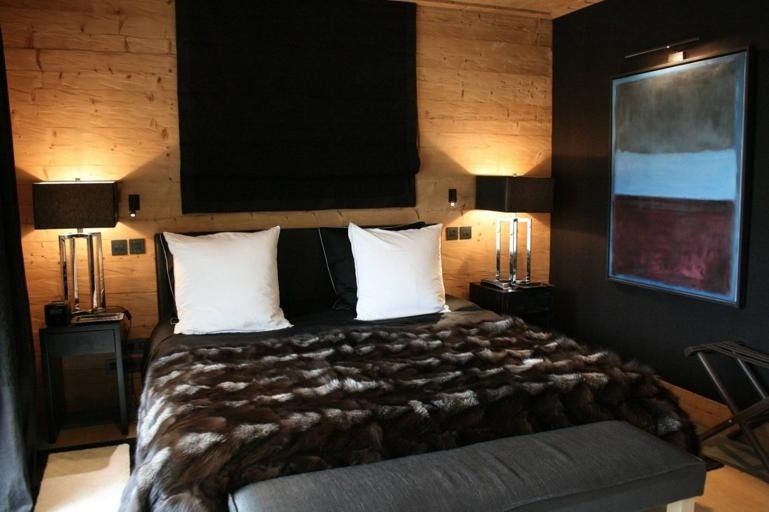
118,223 -> 704,512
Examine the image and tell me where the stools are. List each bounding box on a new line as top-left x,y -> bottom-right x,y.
684,338 -> 769,474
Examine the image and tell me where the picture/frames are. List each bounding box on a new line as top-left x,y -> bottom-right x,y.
605,46 -> 751,305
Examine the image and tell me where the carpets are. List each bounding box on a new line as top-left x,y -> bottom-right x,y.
29,438 -> 137,512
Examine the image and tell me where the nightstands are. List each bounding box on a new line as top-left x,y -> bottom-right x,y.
473,281 -> 574,332
41,313 -> 133,436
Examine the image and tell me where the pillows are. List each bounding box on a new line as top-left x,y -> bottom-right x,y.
157,221 -> 448,332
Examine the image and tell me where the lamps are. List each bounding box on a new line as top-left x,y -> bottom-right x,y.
468,171 -> 560,288
31,179 -> 121,312
